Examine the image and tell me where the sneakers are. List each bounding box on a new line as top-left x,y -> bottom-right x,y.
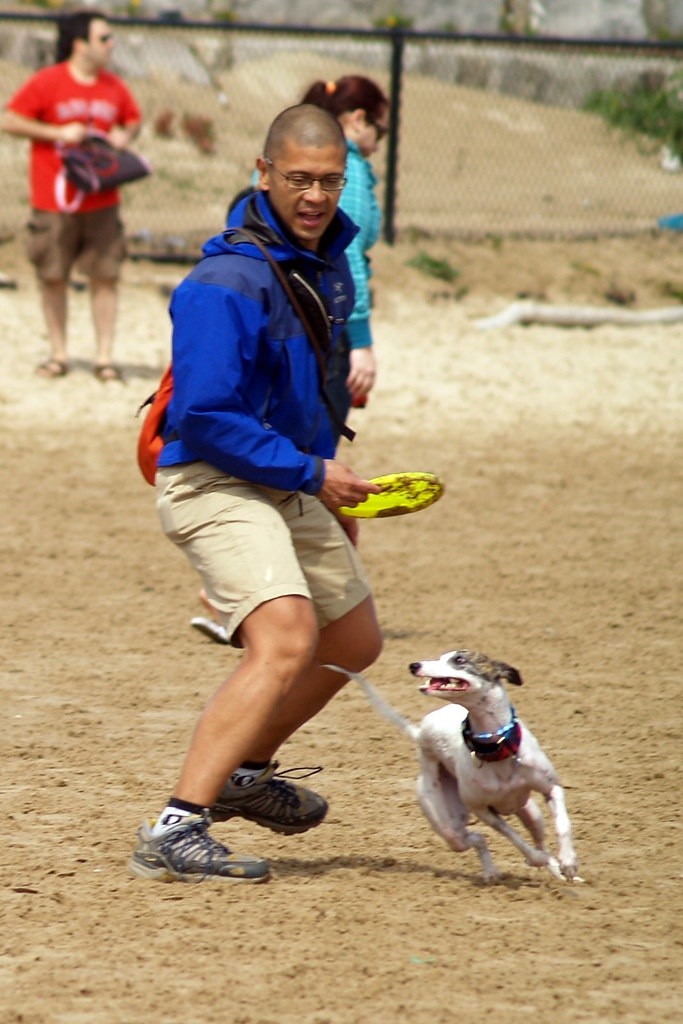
210,760 -> 329,836
127,808 -> 271,884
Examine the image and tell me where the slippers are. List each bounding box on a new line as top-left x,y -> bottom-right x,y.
191,617 -> 229,644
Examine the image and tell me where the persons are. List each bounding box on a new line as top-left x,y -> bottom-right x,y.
192,75 -> 391,649
127,104 -> 384,883
0,10 -> 143,384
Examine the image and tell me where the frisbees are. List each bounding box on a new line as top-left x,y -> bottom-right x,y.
337,472 -> 446,518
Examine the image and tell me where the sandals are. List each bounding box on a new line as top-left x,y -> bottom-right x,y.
36,359 -> 68,376
93,364 -> 125,385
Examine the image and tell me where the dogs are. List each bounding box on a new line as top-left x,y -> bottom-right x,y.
319,648 -> 585,881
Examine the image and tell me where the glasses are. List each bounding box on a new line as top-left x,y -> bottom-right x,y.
364,113 -> 390,141
263,157 -> 347,191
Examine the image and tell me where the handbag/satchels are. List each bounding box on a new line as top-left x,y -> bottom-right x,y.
135,363 -> 174,487
55,134 -> 151,214
